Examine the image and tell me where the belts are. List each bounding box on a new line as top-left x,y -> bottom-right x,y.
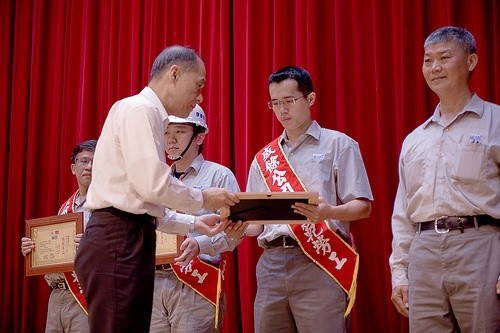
53,281 -> 69,290
416,214 -> 500,233
156,260 -> 219,271
264,235 -> 299,249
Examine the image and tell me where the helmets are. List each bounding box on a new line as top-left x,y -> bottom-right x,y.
169,104 -> 208,134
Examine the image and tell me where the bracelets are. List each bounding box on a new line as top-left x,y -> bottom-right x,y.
189,214 -> 195,233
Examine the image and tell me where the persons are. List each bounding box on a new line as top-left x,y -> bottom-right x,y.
20,45 -> 241,333
224,65 -> 375,332
388,25 -> 499,332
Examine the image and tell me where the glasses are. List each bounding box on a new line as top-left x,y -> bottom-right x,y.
267,95 -> 306,109
74,157 -> 93,166
